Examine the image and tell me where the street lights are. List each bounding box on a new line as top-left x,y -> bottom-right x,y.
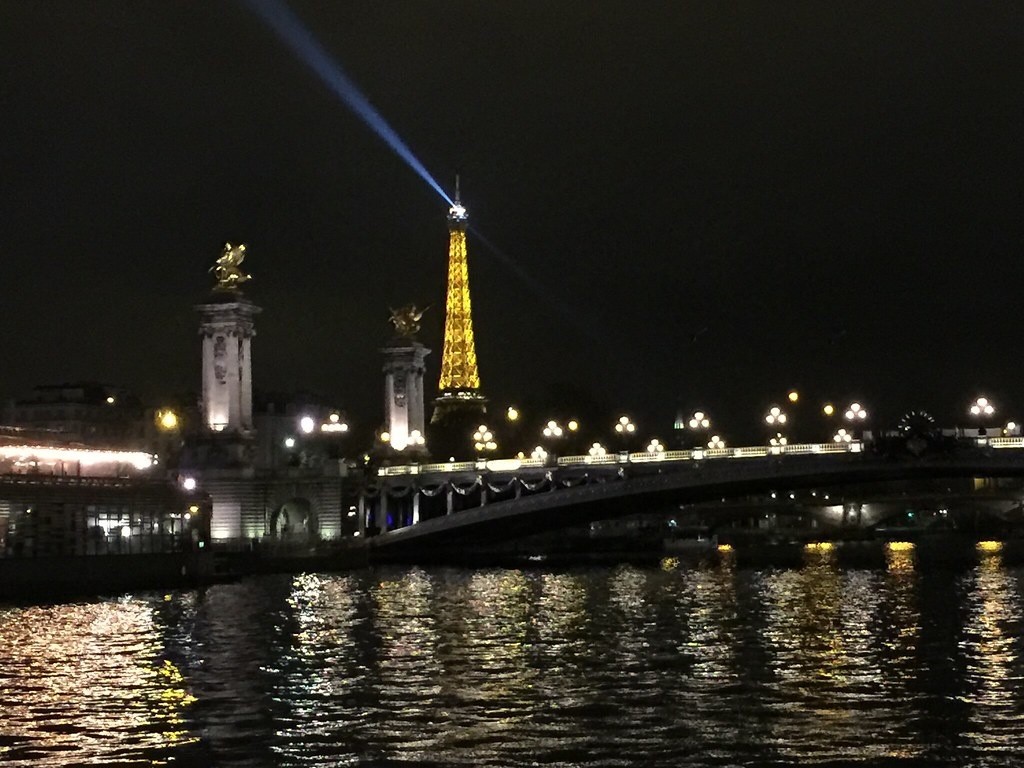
971,398 -> 994,428
690,412 -> 710,448
845,403 -> 866,441
543,421 -> 561,456
615,416 -> 635,454
473,425 -> 493,461
407,430 -> 425,465
766,407 -> 787,446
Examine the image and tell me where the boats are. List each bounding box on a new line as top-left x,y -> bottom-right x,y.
663,526 -> 719,555
